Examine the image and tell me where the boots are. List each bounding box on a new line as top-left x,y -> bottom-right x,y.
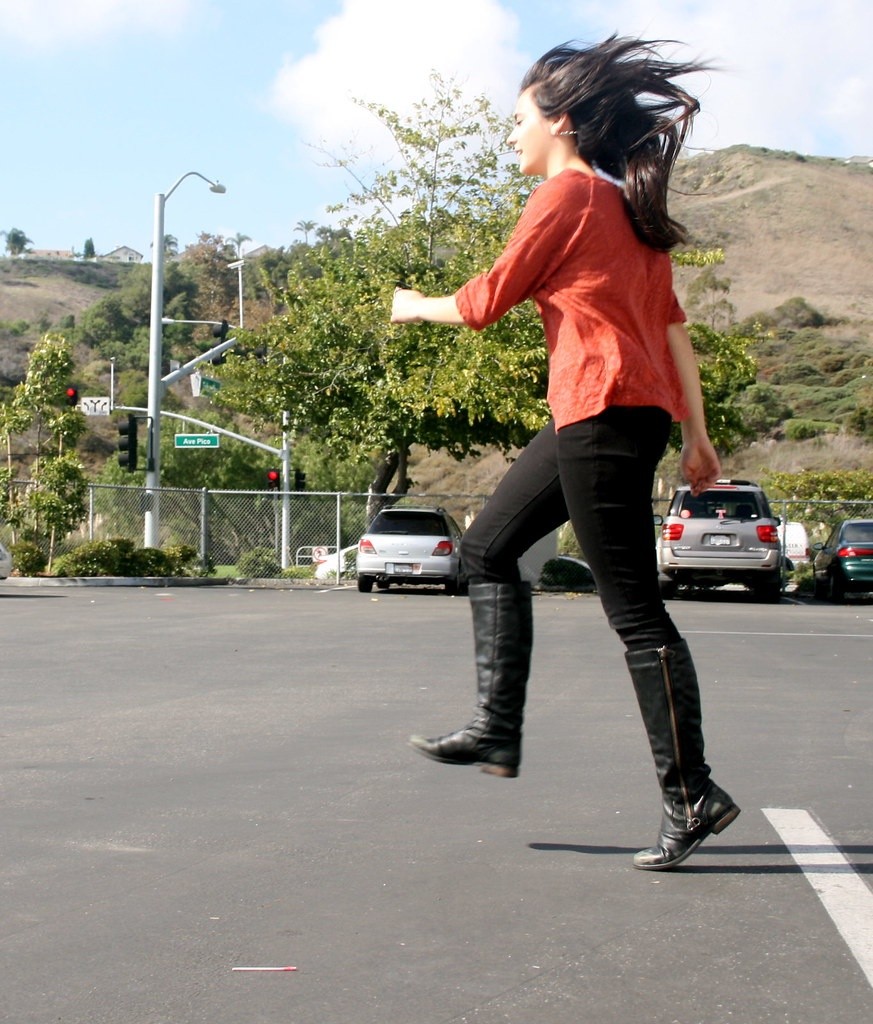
408,579 -> 533,777
626,638 -> 742,869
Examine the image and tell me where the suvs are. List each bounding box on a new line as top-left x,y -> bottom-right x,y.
652,478 -> 782,606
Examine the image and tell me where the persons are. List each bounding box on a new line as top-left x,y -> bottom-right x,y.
390,30 -> 740,872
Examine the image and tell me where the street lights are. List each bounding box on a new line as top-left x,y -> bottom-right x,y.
144,171 -> 227,550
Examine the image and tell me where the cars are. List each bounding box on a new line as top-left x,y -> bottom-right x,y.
810,519 -> 873,607
355,504 -> 468,598
313,545 -> 359,581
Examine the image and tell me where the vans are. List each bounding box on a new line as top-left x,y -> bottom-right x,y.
774,521 -> 810,571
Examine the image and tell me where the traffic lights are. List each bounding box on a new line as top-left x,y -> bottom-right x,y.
209,319 -> 229,366
267,469 -> 280,500
116,413 -> 137,472
295,469 -> 307,491
64,383 -> 79,408
